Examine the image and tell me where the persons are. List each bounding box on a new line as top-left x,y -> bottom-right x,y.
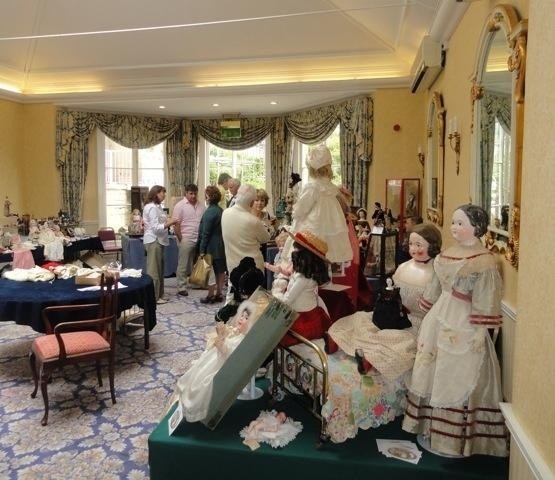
172,184 -> 205,296
196,173 -> 274,303
176,144 -> 508,458
143,186 -> 179,304
263,145 -> 359,346
177,300 -> 255,422
129,209 -> 143,235
322,203 -> 504,458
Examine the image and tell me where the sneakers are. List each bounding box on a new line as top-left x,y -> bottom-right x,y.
178,290 -> 189,296
156,298 -> 169,304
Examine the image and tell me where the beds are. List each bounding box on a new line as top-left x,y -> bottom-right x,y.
274,328 -> 416,440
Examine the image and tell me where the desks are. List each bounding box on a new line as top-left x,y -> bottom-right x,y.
124,230 -> 179,277
262,247 -> 276,288
1,228 -> 100,265
146,382 -> 484,479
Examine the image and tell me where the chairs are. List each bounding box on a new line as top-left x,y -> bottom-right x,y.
29,269 -> 125,428
100,227 -> 118,243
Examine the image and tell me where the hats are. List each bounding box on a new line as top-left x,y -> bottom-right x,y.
304,142 -> 333,172
287,228 -> 334,263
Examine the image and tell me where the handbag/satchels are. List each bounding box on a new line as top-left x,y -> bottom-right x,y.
187,254 -> 214,288
98,226 -> 116,241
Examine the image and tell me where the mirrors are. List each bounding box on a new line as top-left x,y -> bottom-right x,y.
424,89 -> 445,228
467,7 -> 525,272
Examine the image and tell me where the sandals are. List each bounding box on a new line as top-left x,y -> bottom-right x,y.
199,293 -> 224,304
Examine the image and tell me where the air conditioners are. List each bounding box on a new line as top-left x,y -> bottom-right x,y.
408,39 -> 444,97
